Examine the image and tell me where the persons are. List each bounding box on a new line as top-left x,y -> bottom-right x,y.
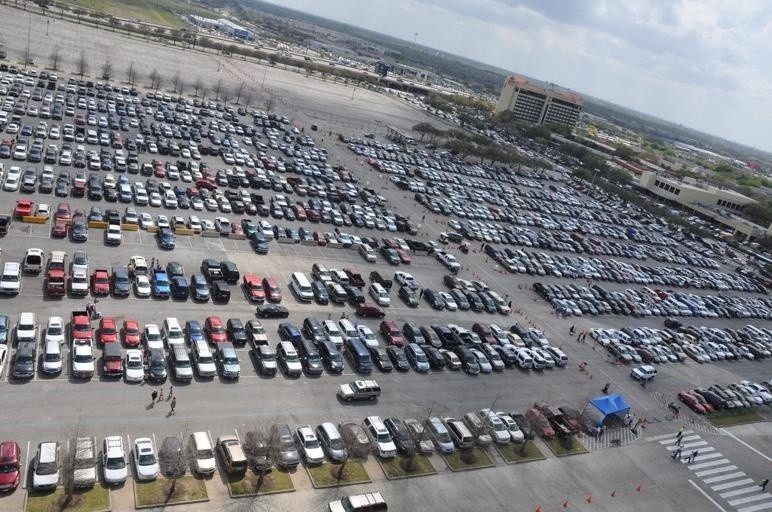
685,451 -> 694,463
601,382 -> 610,393
668,401 -> 681,418
568,324 -> 625,371
691,451 -> 698,462
168,386 -> 173,398
170,397 -> 177,414
151,389 -> 158,403
571,271 -> 594,286
139,346 -> 155,385
544,292 -> 568,319
674,435 -> 684,446
670,450 -> 675,457
502,294 -> 508,301
673,444 -> 684,459
759,478 -> 769,491
84,296 -> 100,319
158,388 -> 165,401
508,299 -> 512,309
676,425 -> 685,438
588,411 -> 646,440
145,256 -> 162,273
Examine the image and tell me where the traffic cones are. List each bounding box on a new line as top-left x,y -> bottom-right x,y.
535,484 -> 642,512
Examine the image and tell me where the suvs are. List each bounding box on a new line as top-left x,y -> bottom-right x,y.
328,491 -> 388,512
